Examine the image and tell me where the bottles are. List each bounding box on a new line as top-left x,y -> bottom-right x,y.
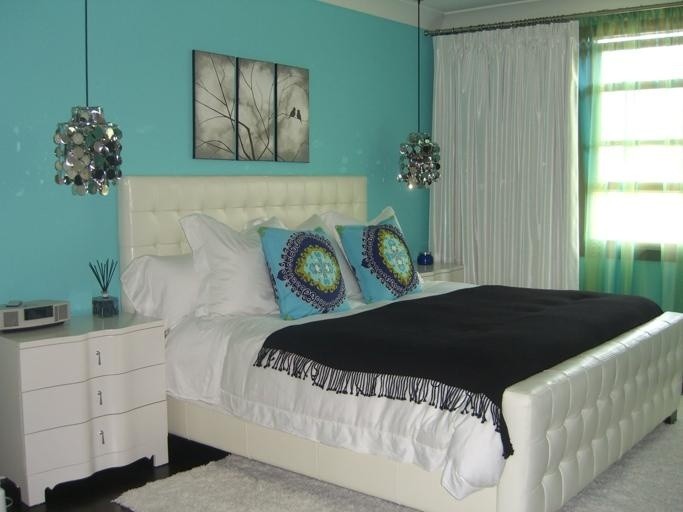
417,252 -> 433,265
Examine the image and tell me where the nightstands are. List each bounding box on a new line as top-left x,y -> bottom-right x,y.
413,265 -> 464,284
0,312 -> 169,507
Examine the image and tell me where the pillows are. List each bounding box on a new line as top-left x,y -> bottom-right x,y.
296,206 -> 423,301
179,213 -> 360,322
334,215 -> 423,304
257,225 -> 350,321
120,215 -> 287,324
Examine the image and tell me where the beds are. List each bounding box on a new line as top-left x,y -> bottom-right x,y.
117,174 -> 683,511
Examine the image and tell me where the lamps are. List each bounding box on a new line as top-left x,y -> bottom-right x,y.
395,0 -> 441,190
55,0 -> 124,197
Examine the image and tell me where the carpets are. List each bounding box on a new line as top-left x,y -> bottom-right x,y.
109,419 -> 683,509
